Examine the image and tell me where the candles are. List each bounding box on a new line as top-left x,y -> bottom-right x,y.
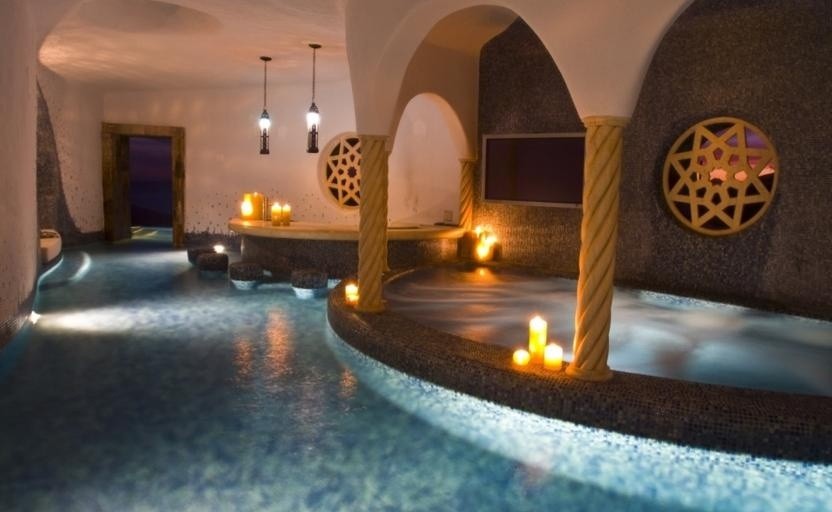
544,342 -> 563,371
240,193 -> 263,220
513,348 -> 531,366
344,282 -> 358,307
271,202 -> 281,226
283,204 -> 293,226
528,315 -> 548,364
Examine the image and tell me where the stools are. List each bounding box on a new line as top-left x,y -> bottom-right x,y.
196,253 -> 229,281
187,245 -> 216,268
230,262 -> 264,291
291,268 -> 329,300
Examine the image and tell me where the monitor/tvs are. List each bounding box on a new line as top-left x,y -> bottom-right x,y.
481,133 -> 587,208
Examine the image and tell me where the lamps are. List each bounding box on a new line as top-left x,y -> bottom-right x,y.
258,56 -> 272,154
307,44 -> 322,153
